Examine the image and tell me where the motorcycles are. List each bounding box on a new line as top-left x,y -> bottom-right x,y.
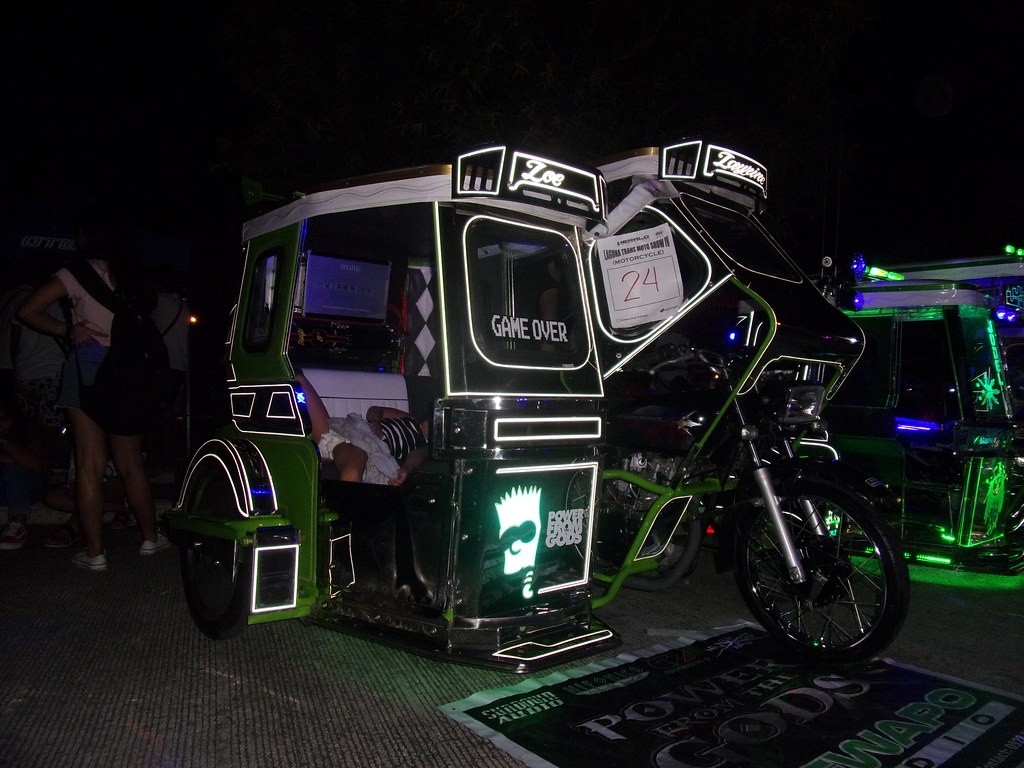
157,139 -> 910,676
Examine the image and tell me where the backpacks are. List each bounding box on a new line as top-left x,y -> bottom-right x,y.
45,251 -> 174,410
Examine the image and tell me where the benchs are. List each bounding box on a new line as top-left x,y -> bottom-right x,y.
303,368 -> 408,421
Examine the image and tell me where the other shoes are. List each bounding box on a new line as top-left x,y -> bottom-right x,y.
110,512 -> 137,530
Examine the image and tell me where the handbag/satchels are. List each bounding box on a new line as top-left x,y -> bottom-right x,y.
37,313 -> 81,427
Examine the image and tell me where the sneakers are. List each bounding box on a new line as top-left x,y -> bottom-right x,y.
44,518 -> 81,547
139,527 -> 171,556
66,547 -> 108,571
0,520 -> 28,549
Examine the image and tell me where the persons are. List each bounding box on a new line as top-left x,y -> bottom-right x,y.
0,205 -> 173,572
293,373 -> 432,486
